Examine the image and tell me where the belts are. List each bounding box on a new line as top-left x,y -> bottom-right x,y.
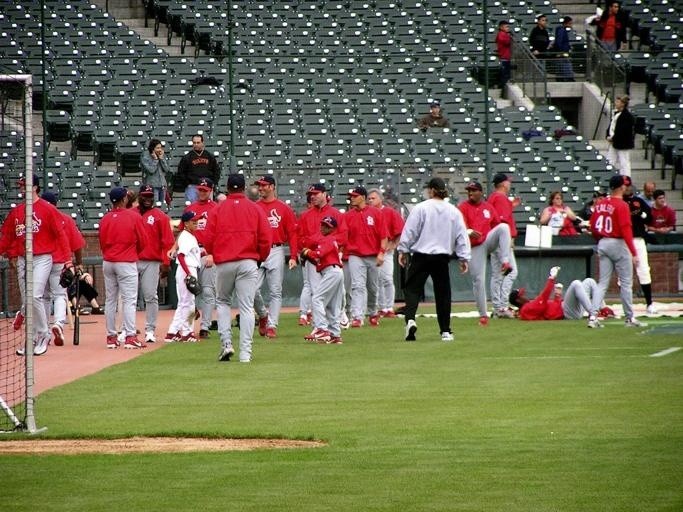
271,244 -> 281,248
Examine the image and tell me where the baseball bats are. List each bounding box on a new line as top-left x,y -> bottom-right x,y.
74,276 -> 79,344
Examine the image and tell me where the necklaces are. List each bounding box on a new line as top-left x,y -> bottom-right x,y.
192,150 -> 204,157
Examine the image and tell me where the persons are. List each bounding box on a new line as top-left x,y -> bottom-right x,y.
211,168 -> 273,364
1,172 -> 676,357
494,21 -> 512,99
176,134 -> 220,205
554,16 -> 575,80
528,12 -> 550,83
417,96 -> 450,130
588,1 -> 636,57
605,94 -> 634,176
139,138 -> 169,210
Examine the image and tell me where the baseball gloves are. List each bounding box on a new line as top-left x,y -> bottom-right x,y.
58,267 -> 74,287
300,248 -> 310,266
184,275 -> 202,296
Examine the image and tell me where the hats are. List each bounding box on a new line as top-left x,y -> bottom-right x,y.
15,173 -> 40,186
494,174 -> 513,184
228,173 -> 245,190
181,210 -> 203,222
320,215 -> 337,228
194,177 -> 213,192
465,182 -> 482,191
110,185 -> 154,203
423,178 -> 446,190
509,287 -> 526,305
306,183 -> 325,194
609,175 -> 631,188
347,187 -> 367,196
254,176 -> 275,185
42,191 -> 58,203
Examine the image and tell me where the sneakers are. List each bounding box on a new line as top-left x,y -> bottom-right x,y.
404,319 -> 418,340
164,331 -> 208,344
625,317 -> 648,328
218,342 -> 235,361
646,304 -> 661,315
587,308 -> 614,329
492,306 -> 519,318
298,308 -> 398,345
441,331 -> 453,341
258,311 -> 277,339
16,325 -> 64,356
71,305 -> 104,315
479,316 -> 488,325
501,262 -> 512,276
12,311 -> 25,332
107,330 -> 156,350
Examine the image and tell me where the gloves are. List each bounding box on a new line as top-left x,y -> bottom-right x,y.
550,266 -> 561,277
554,282 -> 564,295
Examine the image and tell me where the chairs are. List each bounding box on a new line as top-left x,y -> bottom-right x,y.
0,1 -> 683,232
